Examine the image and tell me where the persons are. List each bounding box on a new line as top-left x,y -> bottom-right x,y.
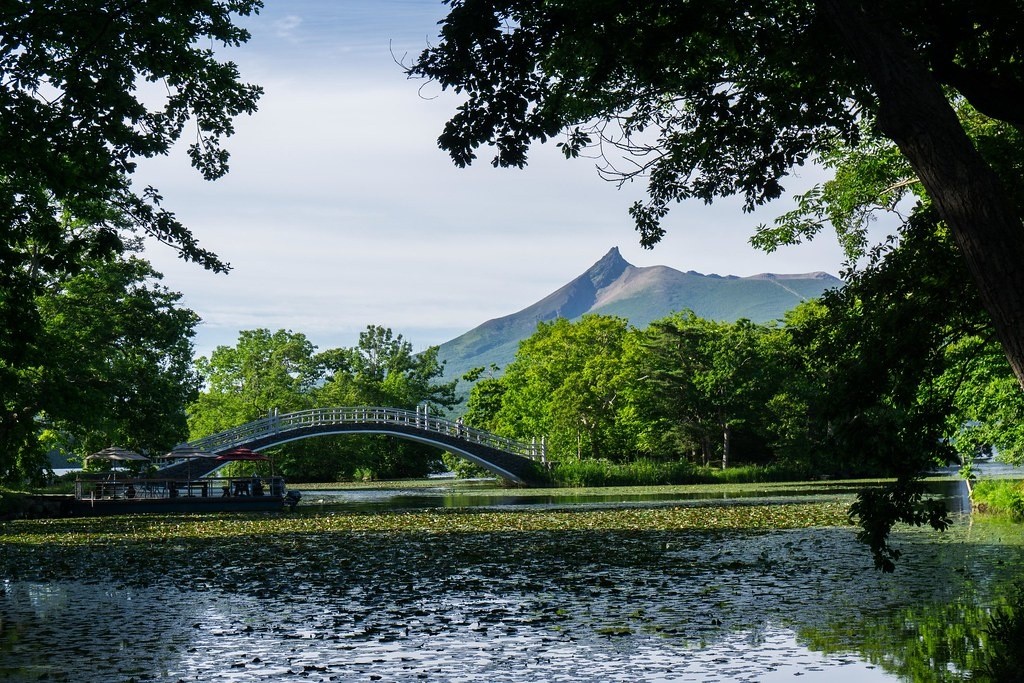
95,480 -> 265,498
455,417 -> 463,435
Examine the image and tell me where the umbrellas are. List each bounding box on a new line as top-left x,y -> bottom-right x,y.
157,445 -> 219,497
86,446 -> 149,498
216,448 -> 270,496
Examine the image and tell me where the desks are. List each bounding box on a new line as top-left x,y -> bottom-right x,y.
172,480 -> 208,497
109,478 -> 174,497
231,480 -> 251,495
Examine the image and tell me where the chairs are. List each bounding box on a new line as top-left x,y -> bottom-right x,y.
95,476 -> 256,498
